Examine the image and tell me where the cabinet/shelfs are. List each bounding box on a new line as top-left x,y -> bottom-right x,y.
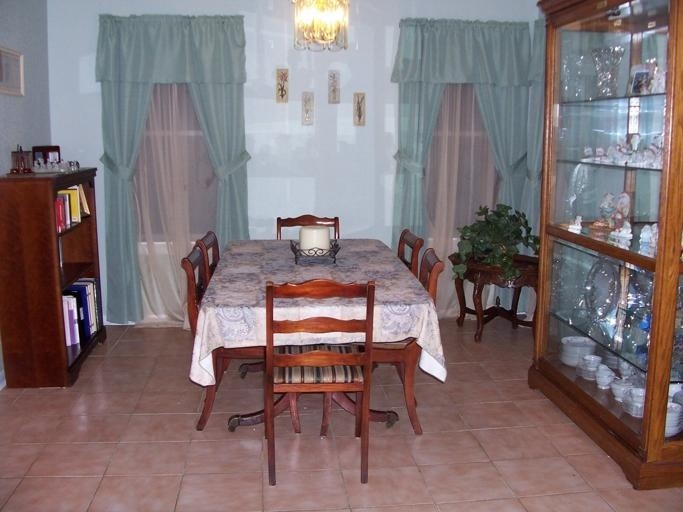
527,0 -> 683,489
0,166 -> 107,388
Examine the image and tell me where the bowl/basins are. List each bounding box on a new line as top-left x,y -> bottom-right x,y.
608,382 -> 634,402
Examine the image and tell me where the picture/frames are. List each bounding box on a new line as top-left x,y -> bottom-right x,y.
0,46 -> 25,96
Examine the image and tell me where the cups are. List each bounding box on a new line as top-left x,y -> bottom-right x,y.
582,356 -> 602,381
595,371 -> 621,390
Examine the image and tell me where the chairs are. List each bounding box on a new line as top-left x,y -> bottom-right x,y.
181,244 -> 300,434
196,231 -> 220,284
264,278 -> 376,488
313,248 -> 445,439
397,229 -> 425,279
275,214 -> 341,240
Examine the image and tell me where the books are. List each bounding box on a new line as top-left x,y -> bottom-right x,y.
58,237 -> 63,269
62,277 -> 101,366
55,184 -> 90,232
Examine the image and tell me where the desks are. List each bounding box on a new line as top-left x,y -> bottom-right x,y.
189,239 -> 446,431
448,252 -> 539,342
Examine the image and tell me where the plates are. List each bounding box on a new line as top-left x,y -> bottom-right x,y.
559,336 -> 596,368
604,353 -> 618,369
665,403 -> 681,439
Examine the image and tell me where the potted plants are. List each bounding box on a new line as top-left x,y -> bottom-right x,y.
452,203 -> 540,282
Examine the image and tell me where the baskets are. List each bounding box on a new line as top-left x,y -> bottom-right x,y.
290,239 -> 341,264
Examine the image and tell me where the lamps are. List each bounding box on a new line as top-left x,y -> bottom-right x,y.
292,1 -> 351,52
